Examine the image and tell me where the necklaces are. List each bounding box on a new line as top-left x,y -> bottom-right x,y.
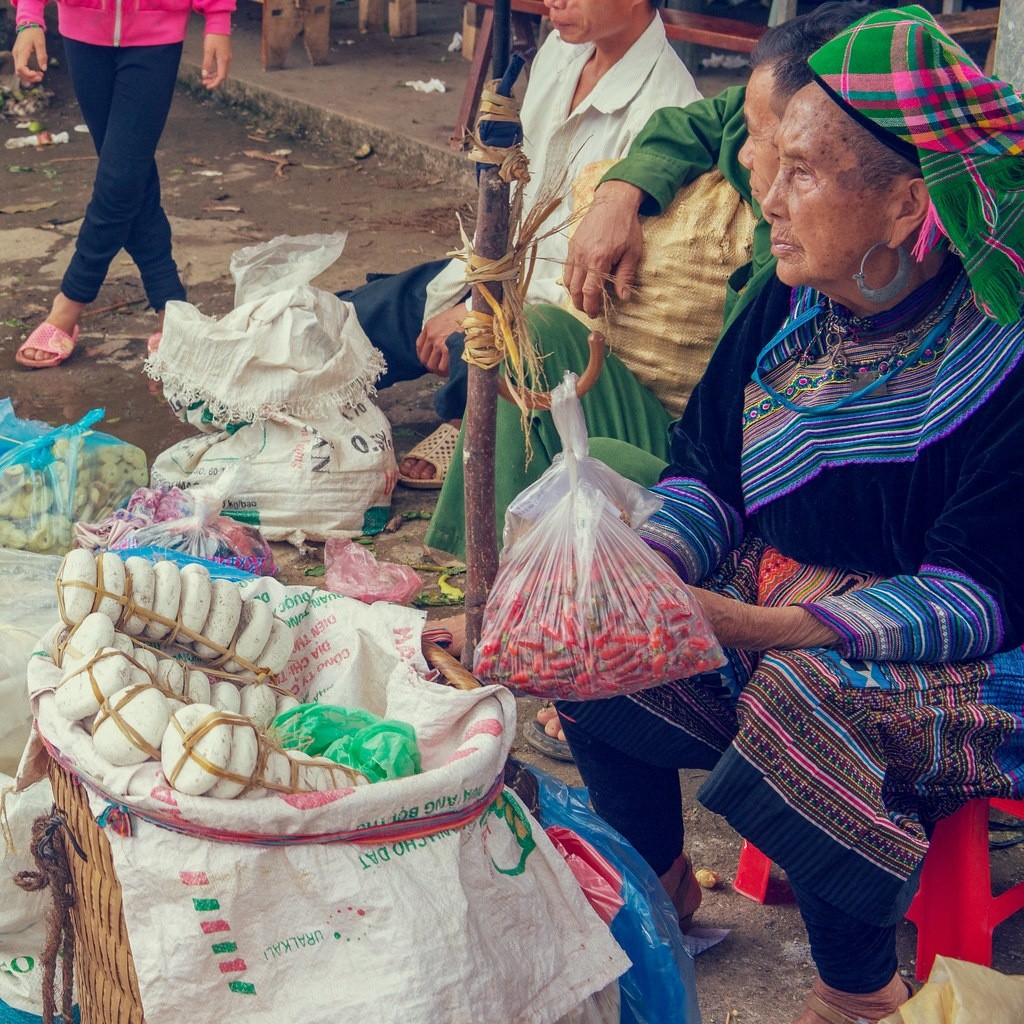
751,267 -> 970,414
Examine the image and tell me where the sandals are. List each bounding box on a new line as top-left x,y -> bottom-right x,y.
670,851 -> 695,932
802,977 -> 915,1024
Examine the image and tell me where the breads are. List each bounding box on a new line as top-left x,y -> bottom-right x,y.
55,549 -> 367,800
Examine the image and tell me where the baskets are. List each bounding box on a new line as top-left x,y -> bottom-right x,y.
27,581 -> 503,1024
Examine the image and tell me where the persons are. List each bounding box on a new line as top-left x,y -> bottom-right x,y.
2,0 -> 235,370
334,0 -> 707,492
548,2 -> 1024,1024
427,1 -> 906,762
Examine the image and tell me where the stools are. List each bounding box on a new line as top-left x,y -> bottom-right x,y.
731,797 -> 1024,985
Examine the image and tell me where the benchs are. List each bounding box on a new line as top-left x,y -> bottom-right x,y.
453,0 -> 771,152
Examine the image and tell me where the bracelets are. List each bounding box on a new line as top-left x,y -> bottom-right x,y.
15,20 -> 47,32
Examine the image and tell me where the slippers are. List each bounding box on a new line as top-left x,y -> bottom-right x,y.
147,333 -> 161,353
397,423 -> 459,488
16,322 -> 79,367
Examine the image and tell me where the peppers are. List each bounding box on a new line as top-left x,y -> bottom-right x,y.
472,555 -> 719,700
